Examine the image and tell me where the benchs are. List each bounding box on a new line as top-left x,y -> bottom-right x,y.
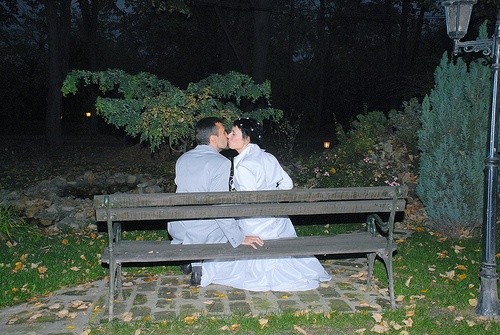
93,186 -> 408,323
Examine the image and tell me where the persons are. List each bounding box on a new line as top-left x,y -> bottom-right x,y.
198,118 -> 294,292
167,118 -> 263,285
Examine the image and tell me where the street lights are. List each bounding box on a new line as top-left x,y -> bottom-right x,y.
442,0 -> 499,318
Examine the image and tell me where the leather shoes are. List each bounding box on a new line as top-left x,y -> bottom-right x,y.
191,272 -> 202,286
181,263 -> 192,275
